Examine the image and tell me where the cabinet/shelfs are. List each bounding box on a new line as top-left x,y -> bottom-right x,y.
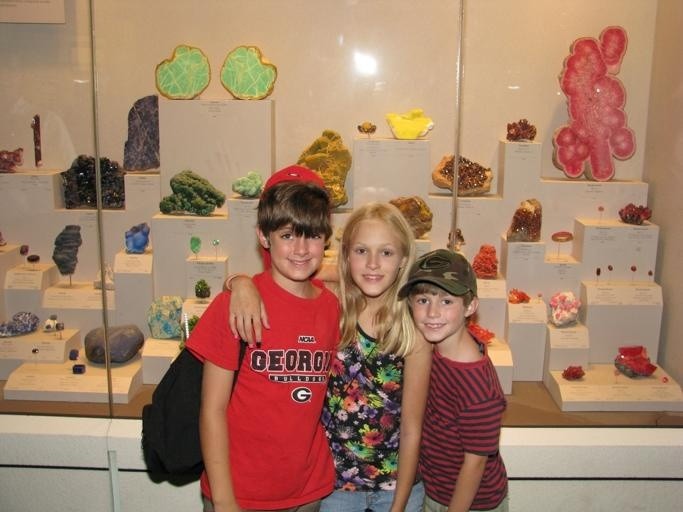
0,99 -> 683,413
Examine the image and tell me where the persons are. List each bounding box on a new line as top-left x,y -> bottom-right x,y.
224,203 -> 433,512
183,167 -> 342,512
396,250 -> 510,511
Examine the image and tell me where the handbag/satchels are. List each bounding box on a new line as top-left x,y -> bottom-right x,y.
142,348 -> 205,486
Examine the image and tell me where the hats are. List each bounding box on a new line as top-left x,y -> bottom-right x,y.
259,166 -> 325,269
398,249 -> 477,297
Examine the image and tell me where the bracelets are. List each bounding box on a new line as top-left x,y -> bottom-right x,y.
224,272 -> 253,292
